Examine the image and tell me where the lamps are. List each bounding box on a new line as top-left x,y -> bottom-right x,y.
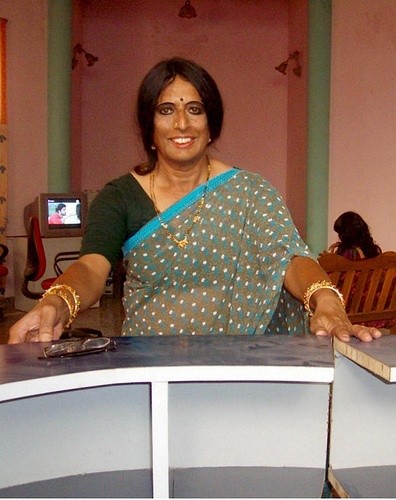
275,50 -> 302,77
73,42 -> 99,69
177,0 -> 198,19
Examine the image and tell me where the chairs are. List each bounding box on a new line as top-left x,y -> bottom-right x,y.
0,243 -> 8,321
21,215 -> 102,339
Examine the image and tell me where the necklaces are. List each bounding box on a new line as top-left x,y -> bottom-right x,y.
150,154 -> 212,249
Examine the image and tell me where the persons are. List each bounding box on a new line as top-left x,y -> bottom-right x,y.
7,57 -> 382,345
48,204 -> 80,225
319,211 -> 396,329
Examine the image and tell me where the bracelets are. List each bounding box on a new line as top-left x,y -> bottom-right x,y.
45,283 -> 81,319
304,278 -> 346,316
39,290 -> 75,328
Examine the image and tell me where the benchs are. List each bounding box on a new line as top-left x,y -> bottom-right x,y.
318,251 -> 396,327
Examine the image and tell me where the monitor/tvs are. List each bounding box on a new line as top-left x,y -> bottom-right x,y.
24,193 -> 88,238
